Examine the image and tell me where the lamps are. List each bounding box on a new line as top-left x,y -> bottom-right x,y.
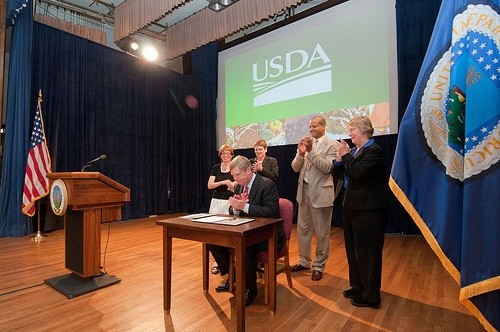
127,37 -> 139,51
208,0 -> 240,13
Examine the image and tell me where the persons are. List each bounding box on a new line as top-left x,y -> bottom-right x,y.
215,155 -> 286,310
248,140 -> 278,187
291,116 -> 341,281
331,116 -> 388,309
207,144 -> 234,274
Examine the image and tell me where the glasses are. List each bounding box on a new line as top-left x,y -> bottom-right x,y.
346,126 -> 360,132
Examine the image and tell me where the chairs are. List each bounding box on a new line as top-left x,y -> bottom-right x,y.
229,198 -> 294,305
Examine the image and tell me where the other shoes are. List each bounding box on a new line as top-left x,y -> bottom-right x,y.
211,259 -> 219,274
344,288 -> 358,298
352,297 -> 380,308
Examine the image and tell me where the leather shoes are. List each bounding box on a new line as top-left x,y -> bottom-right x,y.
216,276 -> 236,292
244,285 -> 258,306
291,264 -> 311,272
312,270 -> 323,281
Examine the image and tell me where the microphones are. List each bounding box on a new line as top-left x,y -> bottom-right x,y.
83,155 -> 107,172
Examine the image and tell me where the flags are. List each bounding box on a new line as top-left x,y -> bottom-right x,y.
385,0 -> 500,332
21,98 -> 53,217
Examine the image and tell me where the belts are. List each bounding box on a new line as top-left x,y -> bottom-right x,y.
303,180 -> 308,184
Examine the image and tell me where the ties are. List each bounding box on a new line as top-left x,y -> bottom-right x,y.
306,140 -> 318,171
243,186 -> 249,200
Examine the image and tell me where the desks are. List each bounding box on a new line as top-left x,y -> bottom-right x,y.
156,213 -> 284,332
